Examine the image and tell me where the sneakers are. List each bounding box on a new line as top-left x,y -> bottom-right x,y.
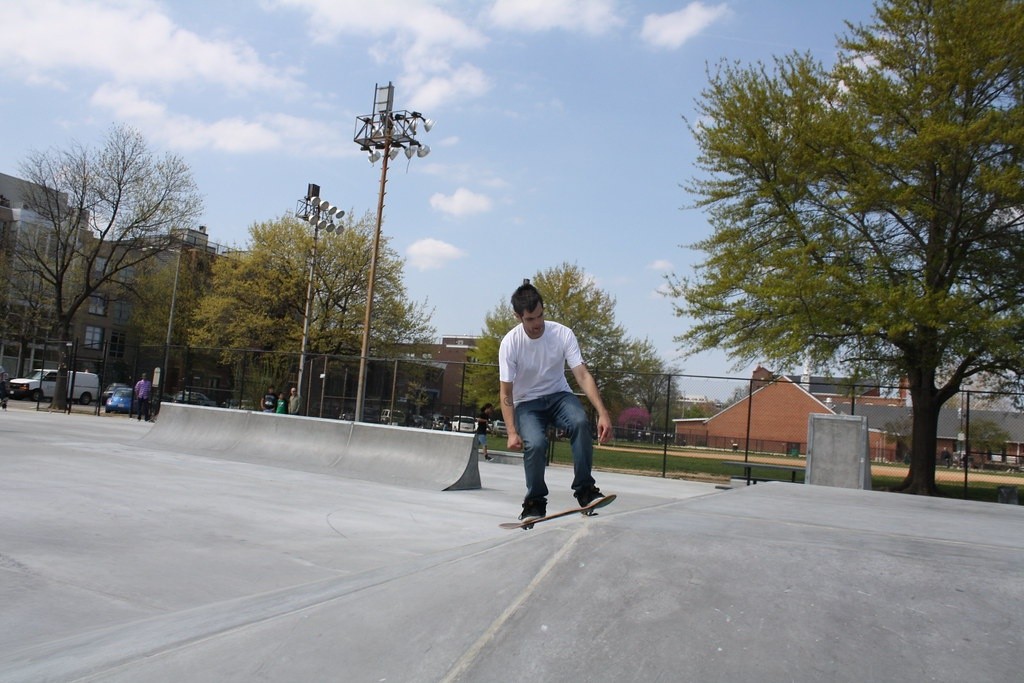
574,485 -> 605,507
519,497 -> 547,522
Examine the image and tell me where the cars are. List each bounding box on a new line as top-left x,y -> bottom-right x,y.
105,387 -> 139,414
626,428 -> 687,446
173,391 -> 217,407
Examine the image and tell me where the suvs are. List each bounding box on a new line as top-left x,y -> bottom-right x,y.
340,405 -> 509,438
102,382 -> 131,406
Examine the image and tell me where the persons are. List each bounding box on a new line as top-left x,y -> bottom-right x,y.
135,373 -> 152,421
261,386 -> 301,415
904,450 -> 910,465
215,391 -> 227,407
499,284 -> 613,522
0,366 -> 7,401
943,447 -> 950,469
48,374 -> 56,381
476,403 -> 494,462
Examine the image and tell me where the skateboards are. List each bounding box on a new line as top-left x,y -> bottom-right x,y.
499,494 -> 617,530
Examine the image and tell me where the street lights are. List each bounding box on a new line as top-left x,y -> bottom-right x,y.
139,246 -> 182,401
352,80 -> 438,423
294,183 -> 346,393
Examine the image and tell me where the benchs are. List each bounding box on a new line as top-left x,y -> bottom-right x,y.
713,476 -> 806,491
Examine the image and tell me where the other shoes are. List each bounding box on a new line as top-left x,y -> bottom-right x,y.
485,455 -> 493,461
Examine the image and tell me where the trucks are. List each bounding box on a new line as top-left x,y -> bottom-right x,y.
8,368 -> 100,406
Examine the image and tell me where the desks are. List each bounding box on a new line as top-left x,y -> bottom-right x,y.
720,459 -> 806,472
